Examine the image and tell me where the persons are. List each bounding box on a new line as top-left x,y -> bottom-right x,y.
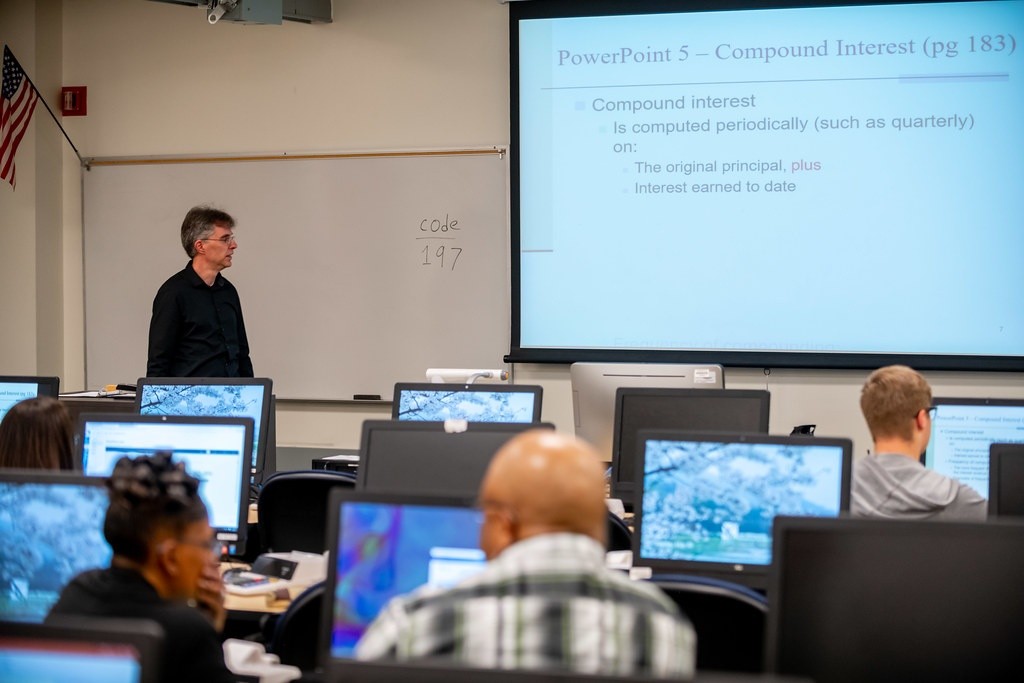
351,427 -> 697,678
0,397 -> 78,472
42,451 -> 273,683
147,204 -> 255,378
850,365 -> 988,524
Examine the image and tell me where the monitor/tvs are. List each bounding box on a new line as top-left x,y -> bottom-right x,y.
0,359 -> 1024,683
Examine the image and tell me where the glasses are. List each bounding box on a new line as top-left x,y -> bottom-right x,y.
916,407 -> 937,420
200,236 -> 235,244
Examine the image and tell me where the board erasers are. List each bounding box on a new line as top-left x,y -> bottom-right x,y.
354,394 -> 381,400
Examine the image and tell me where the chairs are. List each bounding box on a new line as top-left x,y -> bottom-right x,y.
251,469 -> 770,673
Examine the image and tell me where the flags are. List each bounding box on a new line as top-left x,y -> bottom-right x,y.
0,49 -> 38,192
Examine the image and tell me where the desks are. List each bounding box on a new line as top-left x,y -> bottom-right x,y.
59,391 -> 135,421
220,561 -> 309,614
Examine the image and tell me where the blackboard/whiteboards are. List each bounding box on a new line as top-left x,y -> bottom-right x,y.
80,144 -> 511,403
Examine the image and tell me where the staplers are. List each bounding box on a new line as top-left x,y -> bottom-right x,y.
111,383 -> 137,400
